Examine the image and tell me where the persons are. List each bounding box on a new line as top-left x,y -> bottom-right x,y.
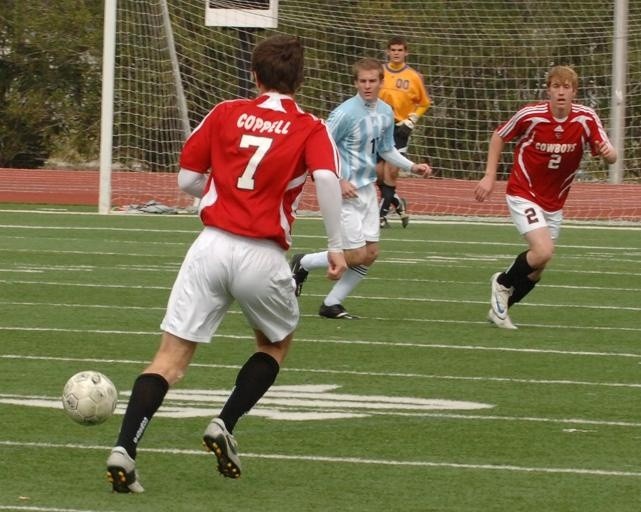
473,65 -> 617,329
374,37 -> 430,229
291,58 -> 431,319
105,34 -> 344,494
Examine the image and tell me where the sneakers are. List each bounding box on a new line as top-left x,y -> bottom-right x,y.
106,445 -> 147,497
400,199 -> 410,228
289,253 -> 309,299
318,301 -> 360,321
486,307 -> 520,331
490,271 -> 513,321
201,418 -> 243,481
380,217 -> 392,229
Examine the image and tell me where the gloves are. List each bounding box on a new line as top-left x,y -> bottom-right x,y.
393,112 -> 421,140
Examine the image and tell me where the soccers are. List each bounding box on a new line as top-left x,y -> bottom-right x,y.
62,370 -> 116,425
